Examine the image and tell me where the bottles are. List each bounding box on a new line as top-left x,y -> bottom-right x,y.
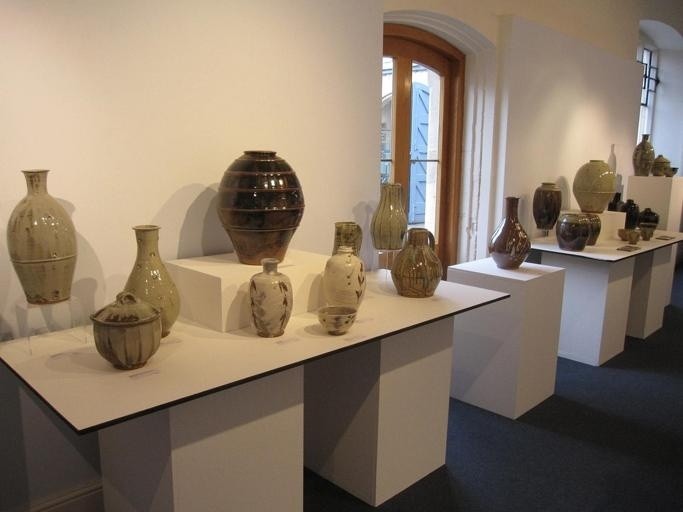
574,157 -> 620,215
611,192 -> 661,231
489,197 -> 531,270
633,135 -> 671,176
532,180 -> 601,253
88,223 -> 180,373
390,229 -> 440,299
321,223 -> 364,310
371,183 -> 406,251
9,168 -> 80,306
213,151 -> 306,261
250,259 -> 290,339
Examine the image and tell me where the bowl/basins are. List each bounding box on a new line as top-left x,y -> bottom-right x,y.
664,167 -> 678,176
618,230 -> 654,245
316,304 -> 356,336
